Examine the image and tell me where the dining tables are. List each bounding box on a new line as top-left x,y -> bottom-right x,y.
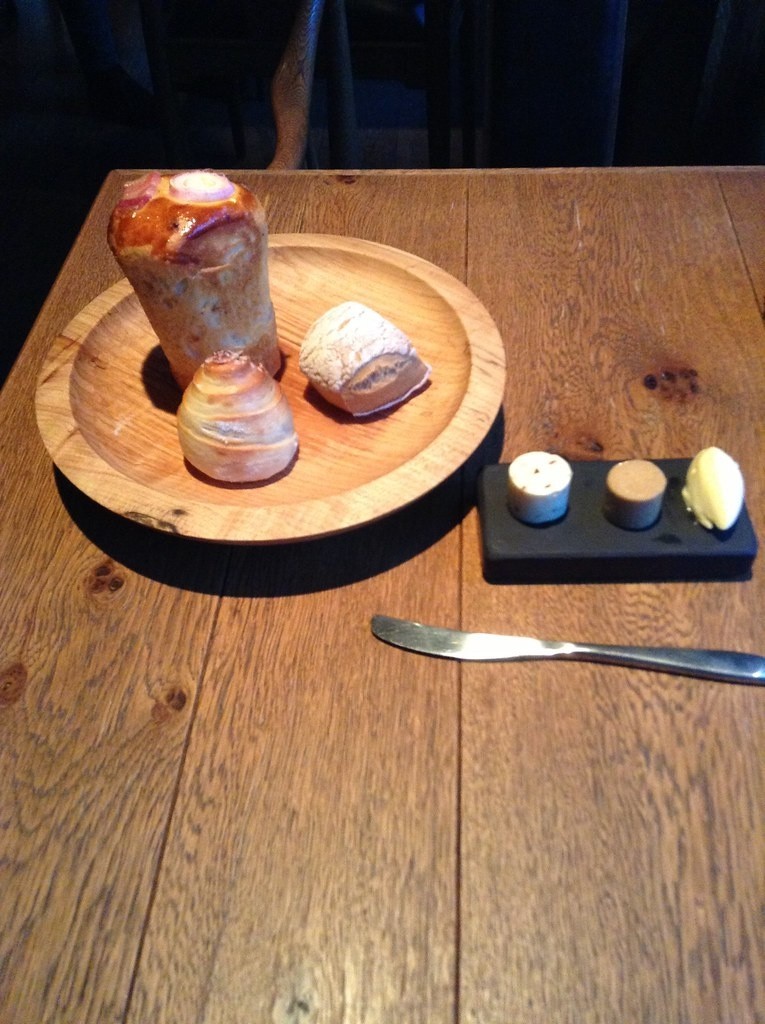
0,169 -> 765,1024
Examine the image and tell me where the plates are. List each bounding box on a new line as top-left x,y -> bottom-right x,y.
35,232 -> 509,543
475,452 -> 759,569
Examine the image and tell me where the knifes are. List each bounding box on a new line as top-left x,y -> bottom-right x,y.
370,612 -> 765,687
